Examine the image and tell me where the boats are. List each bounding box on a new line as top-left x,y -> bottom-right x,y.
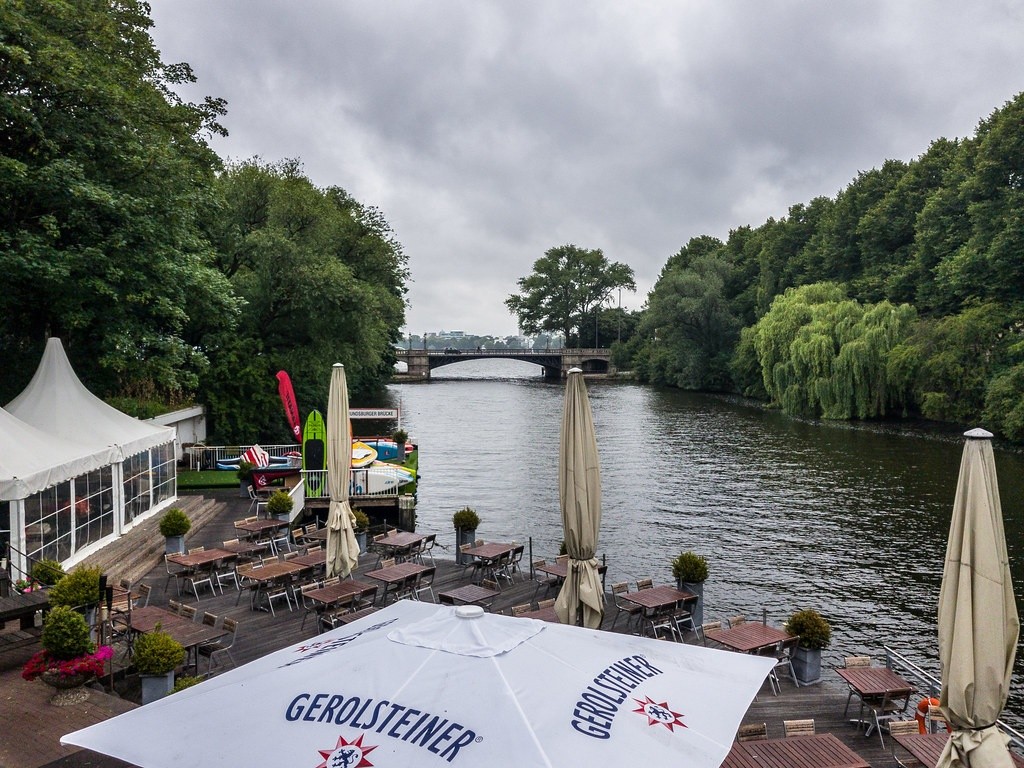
300,409 -> 327,498
350,436 -> 417,496
216,451 -> 302,471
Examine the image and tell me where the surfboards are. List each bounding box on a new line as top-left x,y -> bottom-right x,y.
302,409 -> 416,498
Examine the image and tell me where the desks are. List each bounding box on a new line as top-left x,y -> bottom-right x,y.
236,561 -> 309,612
337,606 -> 380,625
618,585 -> 693,638
361,561 -> 427,606
716,732 -> 872,768
301,525 -> 328,546
293,548 -> 328,585
376,532 -> 427,566
462,542 -> 525,590
255,486 -> 291,518
706,621 -> 793,655
834,667 -> 919,734
219,540 -> 267,583
439,583 -> 501,613
540,557 -> 611,600
302,579 -> 379,621
236,519 -> 289,543
166,546 -> 239,599
891,733 -> 1024,768
108,605 -> 229,677
515,605 -> 562,624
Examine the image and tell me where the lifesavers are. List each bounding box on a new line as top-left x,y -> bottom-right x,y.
914,697 -> 952,735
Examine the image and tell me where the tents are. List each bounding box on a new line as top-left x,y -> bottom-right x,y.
60,597 -> 779,768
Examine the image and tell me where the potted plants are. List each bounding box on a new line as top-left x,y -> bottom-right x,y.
671,549 -> 709,629
236,459 -> 257,498
41,605 -> 100,707
129,621 -> 186,708
157,508 -> 192,556
351,510 -> 370,555
393,428 -> 408,460
267,488 -> 295,538
47,561 -> 107,643
786,607 -> 834,683
452,506 -> 482,566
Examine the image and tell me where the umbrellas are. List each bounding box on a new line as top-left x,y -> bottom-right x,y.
553,366 -> 609,630
326,363 -> 361,580
933,426 -> 1020,768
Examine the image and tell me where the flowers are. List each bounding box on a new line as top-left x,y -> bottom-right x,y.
15,574 -> 42,593
21,644 -> 114,683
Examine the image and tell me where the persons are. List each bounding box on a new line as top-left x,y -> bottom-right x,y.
62,495 -> 93,518
476,346 -> 482,353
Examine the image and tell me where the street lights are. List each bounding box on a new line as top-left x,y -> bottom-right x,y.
546,334 -> 549,349
617,288 -> 622,343
559,335 -> 561,348
408,333 -> 412,349
424,333 -> 428,349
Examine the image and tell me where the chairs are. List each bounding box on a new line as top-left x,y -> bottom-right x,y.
99,484 -> 954,743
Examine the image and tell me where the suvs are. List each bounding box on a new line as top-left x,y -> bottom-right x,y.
443,347 -> 462,355
